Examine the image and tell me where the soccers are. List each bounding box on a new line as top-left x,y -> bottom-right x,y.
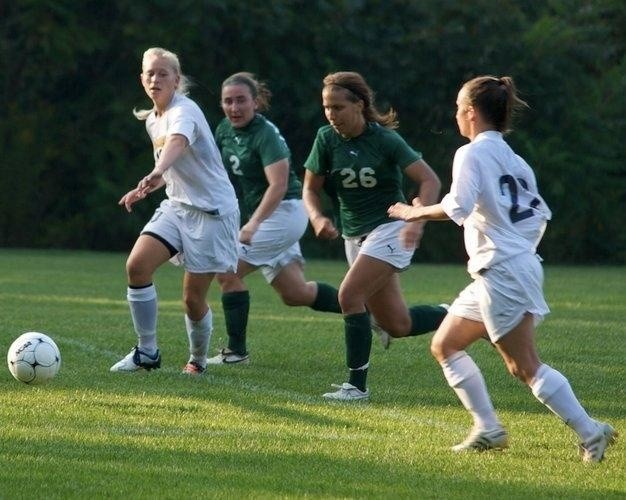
7,333 -> 64,384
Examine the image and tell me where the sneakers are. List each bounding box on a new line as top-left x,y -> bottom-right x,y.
578,423 -> 618,462
109,344 -> 163,373
367,310 -> 390,350
452,425 -> 507,451
206,347 -> 250,365
182,363 -> 207,375
321,381 -> 370,402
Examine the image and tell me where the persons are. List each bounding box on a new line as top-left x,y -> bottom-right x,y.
110,47 -> 241,374
386,75 -> 618,464
302,72 -> 451,401
206,71 -> 392,367
